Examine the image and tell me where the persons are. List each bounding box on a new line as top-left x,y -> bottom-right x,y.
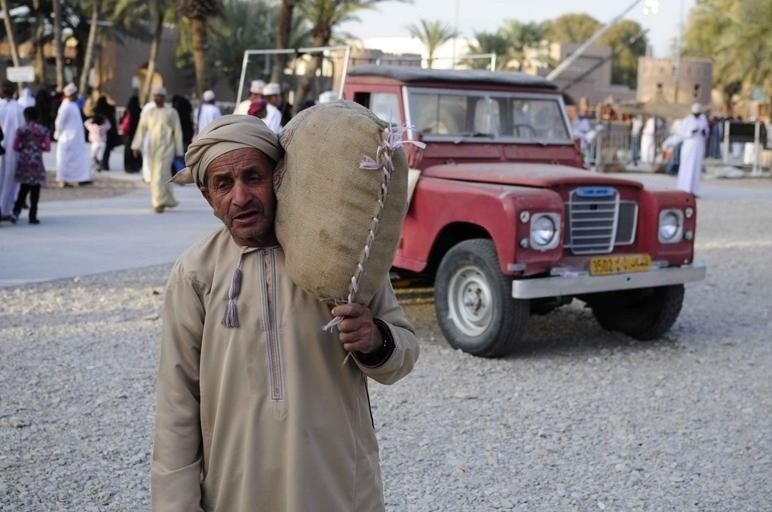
232,79 -> 265,114
571,103 -> 747,199
251,84 -> 285,135
129,86 -> 186,215
171,95 -> 186,177
129,88 -> 142,172
186,99 -> 193,151
193,89 -> 223,139
149,115 -> 420,512
0,72 -> 129,224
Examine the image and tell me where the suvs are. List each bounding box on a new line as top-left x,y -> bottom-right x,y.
341,67 -> 705,358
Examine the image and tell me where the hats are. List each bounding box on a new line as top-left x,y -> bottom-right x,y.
319,91 -> 336,104
263,83 -> 281,96
203,90 -> 216,102
249,79 -> 266,94
154,87 -> 167,96
64,82 -> 77,97
692,104 -> 701,114
